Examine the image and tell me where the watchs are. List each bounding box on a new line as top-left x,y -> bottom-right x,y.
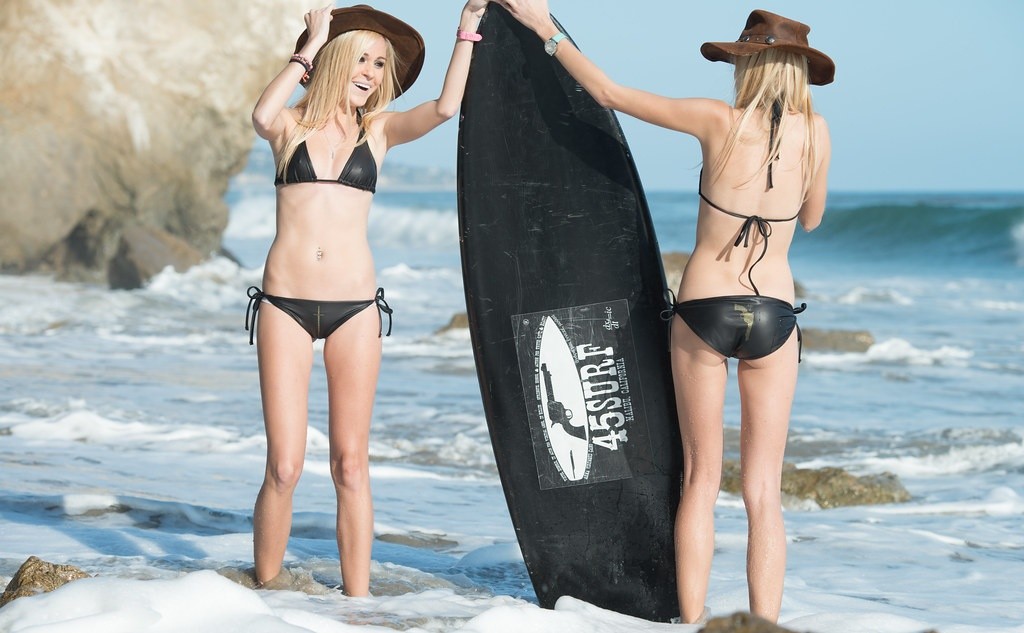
545,32 -> 567,56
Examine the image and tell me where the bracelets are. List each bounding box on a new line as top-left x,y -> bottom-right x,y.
456,27 -> 482,42
289,54 -> 313,82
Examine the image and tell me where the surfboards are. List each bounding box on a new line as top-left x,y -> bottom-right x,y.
452,0 -> 687,623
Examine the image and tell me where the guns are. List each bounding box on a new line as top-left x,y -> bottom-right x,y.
540,361 -> 588,443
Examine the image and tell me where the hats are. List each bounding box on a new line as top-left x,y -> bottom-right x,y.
294,4 -> 426,104
700,9 -> 835,86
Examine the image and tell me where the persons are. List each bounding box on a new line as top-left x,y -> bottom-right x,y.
245,0 -> 489,598
491,0 -> 836,624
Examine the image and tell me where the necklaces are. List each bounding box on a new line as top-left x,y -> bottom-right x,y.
323,128 -> 343,160
